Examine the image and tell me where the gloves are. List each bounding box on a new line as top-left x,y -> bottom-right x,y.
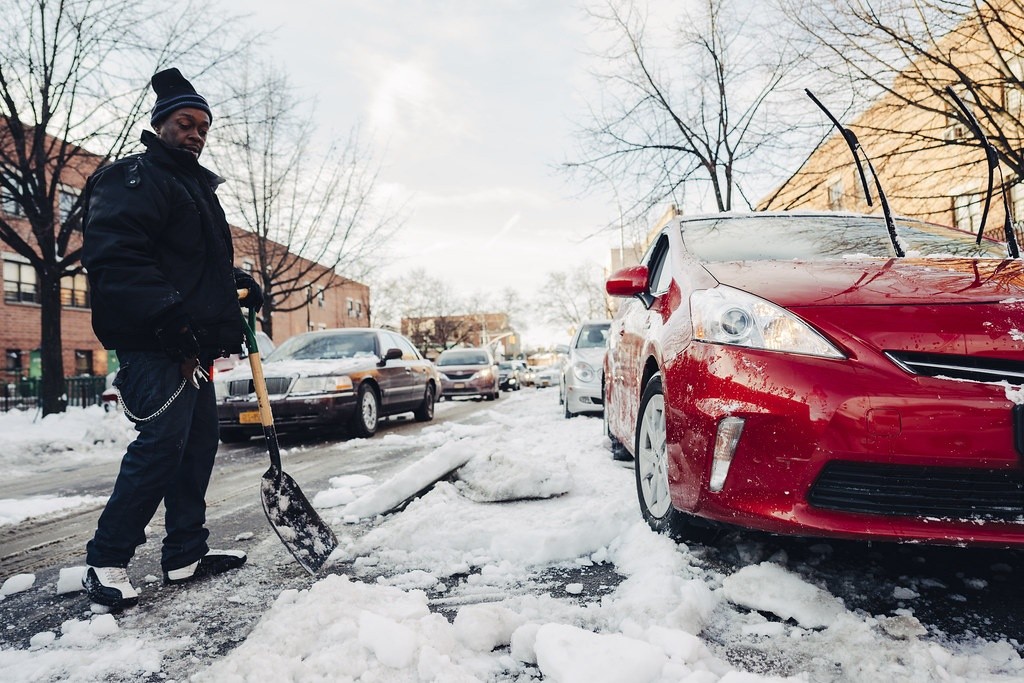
236,274 -> 264,313
144,312 -> 209,359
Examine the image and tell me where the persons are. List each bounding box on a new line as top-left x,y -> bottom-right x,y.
82,67 -> 263,607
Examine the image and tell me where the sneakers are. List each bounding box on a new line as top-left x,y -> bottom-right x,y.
81,564 -> 139,606
163,548 -> 247,585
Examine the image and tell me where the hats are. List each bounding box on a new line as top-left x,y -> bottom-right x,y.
150,67 -> 212,127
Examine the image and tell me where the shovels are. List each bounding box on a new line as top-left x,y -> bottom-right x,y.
238,292 -> 339,573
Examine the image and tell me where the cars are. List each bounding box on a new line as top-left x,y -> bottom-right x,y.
496,350 -> 566,392
102,328 -> 277,412
432,347 -> 500,402
560,319 -> 611,420
214,327 -> 442,443
606,89 -> 1024,553
